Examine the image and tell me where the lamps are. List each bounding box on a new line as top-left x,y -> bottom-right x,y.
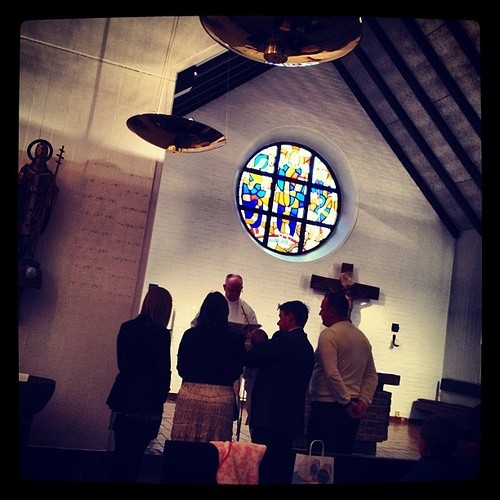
198,14 -> 363,67
126,16 -> 231,154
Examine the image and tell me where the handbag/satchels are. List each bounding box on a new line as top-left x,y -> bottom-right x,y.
292,441 -> 334,484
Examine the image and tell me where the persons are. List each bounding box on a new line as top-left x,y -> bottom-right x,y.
105,288 -> 172,483
171,291 -> 246,442
243,329 -> 268,391
19,143 -> 58,272
327,285 -> 365,323
308,292 -> 377,452
244,301 -> 315,485
190,274 -> 259,345
